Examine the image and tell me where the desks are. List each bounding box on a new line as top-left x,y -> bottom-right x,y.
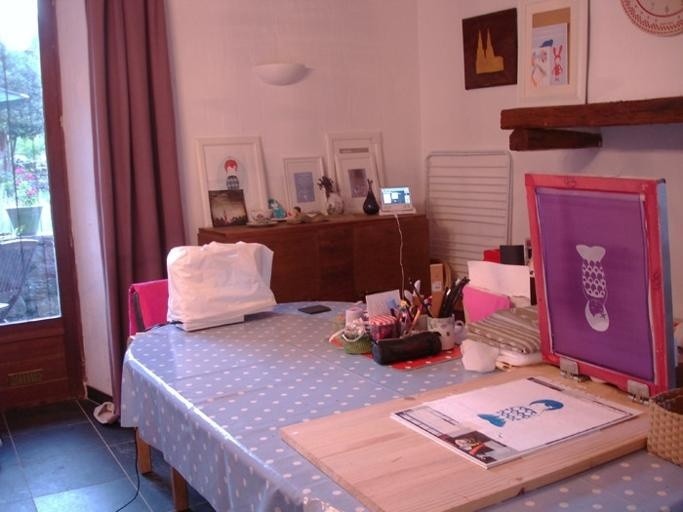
120,299 -> 682,512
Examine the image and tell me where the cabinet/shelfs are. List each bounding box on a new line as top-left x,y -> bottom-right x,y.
197,214 -> 431,308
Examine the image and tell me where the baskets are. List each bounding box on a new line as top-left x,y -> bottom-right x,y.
646,387 -> 683,466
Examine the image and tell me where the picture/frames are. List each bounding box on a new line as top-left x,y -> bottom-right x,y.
197,133 -> 269,228
522,168 -> 677,402
516,0 -> 590,105
280,156 -> 330,221
324,131 -> 388,218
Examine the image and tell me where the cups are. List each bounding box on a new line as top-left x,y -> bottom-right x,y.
426,313 -> 465,350
250,208 -> 272,223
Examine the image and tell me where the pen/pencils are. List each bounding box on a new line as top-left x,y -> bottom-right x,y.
390,304 -> 423,338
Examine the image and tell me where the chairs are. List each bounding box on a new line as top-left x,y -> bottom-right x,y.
1,239 -> 39,324
127,276 -> 207,476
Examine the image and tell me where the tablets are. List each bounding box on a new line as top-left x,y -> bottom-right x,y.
381,185 -> 413,212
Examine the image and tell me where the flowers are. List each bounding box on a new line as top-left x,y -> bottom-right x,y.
7,159 -> 47,203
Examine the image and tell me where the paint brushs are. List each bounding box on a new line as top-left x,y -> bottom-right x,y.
438,275 -> 470,318
408,277 -> 434,318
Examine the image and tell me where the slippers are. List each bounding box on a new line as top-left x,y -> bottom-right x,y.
93,401 -> 116,424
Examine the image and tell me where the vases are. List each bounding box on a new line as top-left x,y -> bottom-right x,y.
4,204 -> 46,240
361,189 -> 379,215
325,191 -> 343,216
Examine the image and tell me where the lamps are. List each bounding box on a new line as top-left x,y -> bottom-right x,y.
252,62 -> 309,87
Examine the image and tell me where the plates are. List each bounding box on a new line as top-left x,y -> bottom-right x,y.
245,221 -> 278,227
